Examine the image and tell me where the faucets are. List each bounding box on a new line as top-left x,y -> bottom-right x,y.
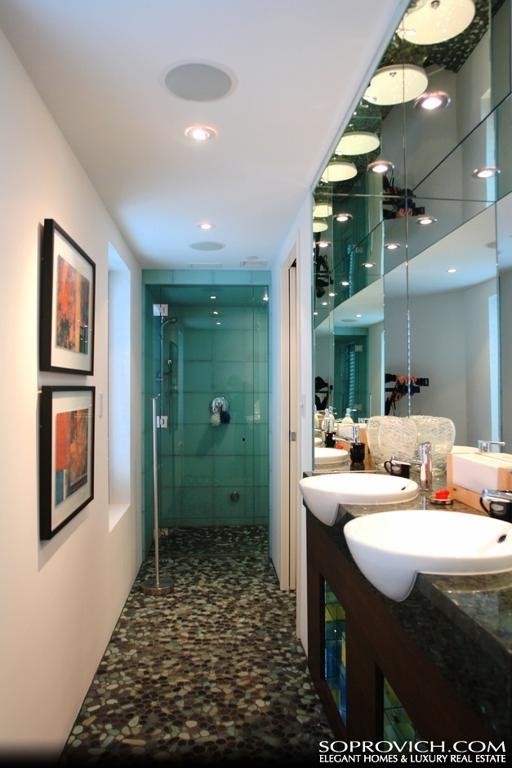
331,425 -> 359,448
480,489 -> 512,503
478,439 -> 505,454
390,442 -> 433,491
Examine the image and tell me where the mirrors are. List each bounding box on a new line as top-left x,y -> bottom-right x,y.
311,1 -> 512,474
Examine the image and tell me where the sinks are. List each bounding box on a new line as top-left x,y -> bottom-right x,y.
298,472 -> 419,527
314,447 -> 348,465
314,437 -> 323,445
343,508 -> 512,603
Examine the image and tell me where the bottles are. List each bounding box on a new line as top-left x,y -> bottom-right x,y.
431,433 -> 448,492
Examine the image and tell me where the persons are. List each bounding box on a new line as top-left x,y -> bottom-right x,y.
315,375 -> 334,411
315,232 -> 334,298
386,372 -> 417,416
382,174 -> 416,220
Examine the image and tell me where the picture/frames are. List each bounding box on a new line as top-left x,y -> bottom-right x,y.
40,218 -> 96,376
37,385 -> 96,541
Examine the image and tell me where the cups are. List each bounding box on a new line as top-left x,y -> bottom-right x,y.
384,459 -> 411,479
325,432 -> 336,447
479,489 -> 512,523
350,460 -> 365,470
350,442 -> 365,463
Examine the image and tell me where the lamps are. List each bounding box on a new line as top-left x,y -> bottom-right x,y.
313,0 -> 475,235
